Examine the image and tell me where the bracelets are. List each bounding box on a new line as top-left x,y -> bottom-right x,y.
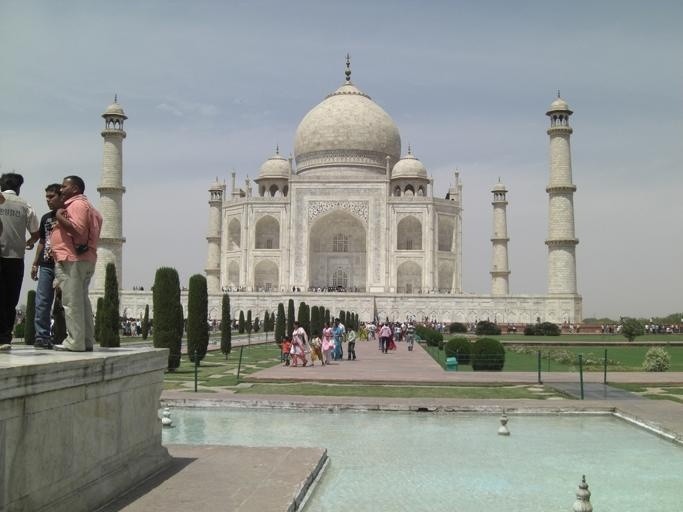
31,265 -> 38,269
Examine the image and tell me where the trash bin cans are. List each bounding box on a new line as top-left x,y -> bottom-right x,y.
444,357 -> 457,371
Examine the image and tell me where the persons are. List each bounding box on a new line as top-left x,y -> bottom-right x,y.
204,319 -> 238,331
404,322 -> 416,351
358,317 -> 448,343
597,321 -> 683,335
512,323 -> 516,334
506,323 -> 510,333
305,287 -> 356,293
117,316 -> 143,338
276,316 -> 356,366
268,286 -> 271,293
28,182 -> 67,349
0,172 -> 41,353
221,283 -> 245,293
47,175 -> 103,353
378,321 -> 392,353
257,286 -> 261,292
555,321 -> 581,334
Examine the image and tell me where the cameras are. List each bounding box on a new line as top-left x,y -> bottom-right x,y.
74,243 -> 89,255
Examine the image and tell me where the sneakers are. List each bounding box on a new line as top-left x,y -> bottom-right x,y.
0,343 -> 11,351
53,343 -> 94,352
34,340 -> 54,351
